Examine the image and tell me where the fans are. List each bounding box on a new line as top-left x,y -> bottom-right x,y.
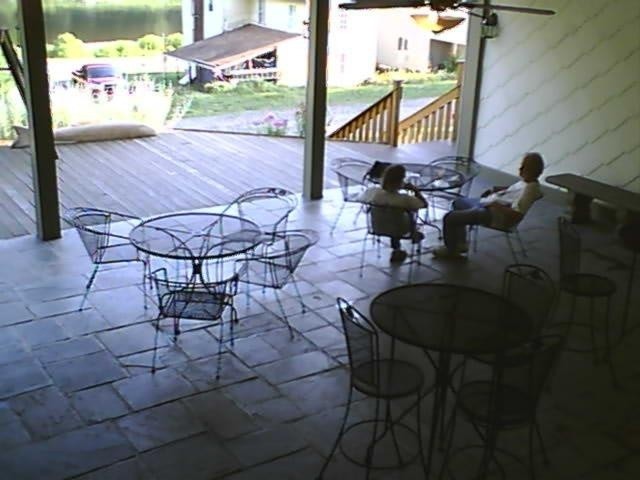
338,0 -> 556,17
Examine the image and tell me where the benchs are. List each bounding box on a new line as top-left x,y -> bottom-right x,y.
544,174 -> 640,237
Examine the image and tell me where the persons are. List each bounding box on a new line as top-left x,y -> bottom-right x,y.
434,153 -> 545,258
359,163 -> 428,262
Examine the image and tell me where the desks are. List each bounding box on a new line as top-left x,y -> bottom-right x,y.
364,162 -> 463,251
372,283 -> 536,476
129,212 -> 261,335
617,221 -> 639,339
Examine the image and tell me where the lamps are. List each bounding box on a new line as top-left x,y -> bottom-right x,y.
479,14 -> 497,39
409,11 -> 465,34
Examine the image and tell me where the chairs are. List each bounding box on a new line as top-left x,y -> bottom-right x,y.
144,268 -> 241,382
202,186 -> 297,294
60,206 -> 153,311
467,188 -> 544,271
318,297 -> 430,479
551,215 -> 620,386
414,156 -> 482,223
456,261 -> 554,398
330,158 -> 377,245
443,341 -> 573,480
359,201 -> 422,286
233,229 -> 321,343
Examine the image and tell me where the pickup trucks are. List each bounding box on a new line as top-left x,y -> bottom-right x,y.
67,62 -> 130,102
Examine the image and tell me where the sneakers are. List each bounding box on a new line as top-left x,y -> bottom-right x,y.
390,249 -> 407,263
433,240 -> 469,259
411,231 -> 425,244
